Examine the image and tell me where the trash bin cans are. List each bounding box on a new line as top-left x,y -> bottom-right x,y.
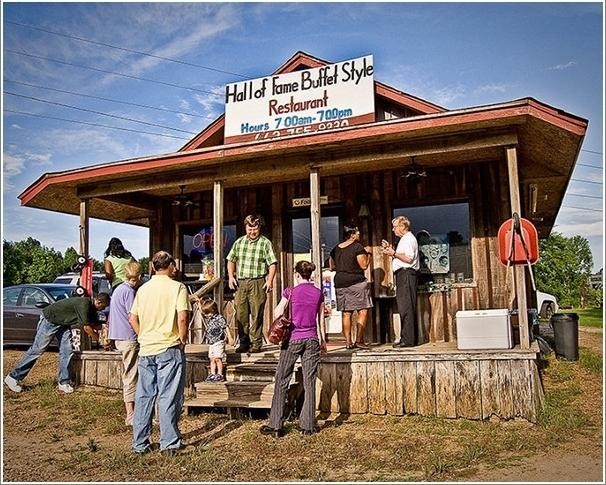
551,312 -> 579,362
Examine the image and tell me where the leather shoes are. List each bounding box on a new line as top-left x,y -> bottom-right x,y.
297,426 -> 311,436
355,341 -> 369,348
235,344 -> 250,353
346,344 -> 353,348
393,340 -> 415,347
396,339 -> 418,346
249,346 -> 264,353
259,425 -> 282,439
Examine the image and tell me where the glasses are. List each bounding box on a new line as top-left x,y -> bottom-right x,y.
393,226 -> 400,228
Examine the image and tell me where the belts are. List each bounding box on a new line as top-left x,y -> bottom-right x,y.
238,277 -> 264,279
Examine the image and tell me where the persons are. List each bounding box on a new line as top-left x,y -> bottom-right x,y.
191,292 -> 237,382
127,250 -> 197,456
4,292 -> 112,394
328,223 -> 375,350
226,213 -> 279,354
106,261 -> 150,428
381,215 -> 423,349
103,237 -> 144,302
258,260 -> 328,440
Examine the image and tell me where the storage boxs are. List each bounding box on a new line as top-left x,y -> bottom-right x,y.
455,309 -> 514,350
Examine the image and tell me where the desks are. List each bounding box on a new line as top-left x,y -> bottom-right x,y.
181,280 -> 238,344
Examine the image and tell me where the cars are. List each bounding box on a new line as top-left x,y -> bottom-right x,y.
535,287 -> 560,319
2,282 -> 103,350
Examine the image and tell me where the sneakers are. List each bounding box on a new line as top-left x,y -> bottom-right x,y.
125,418 -> 133,425
162,443 -> 194,454
5,375 -> 22,392
58,382 -> 74,393
136,442 -> 160,452
212,374 -> 224,381
205,374 -> 214,381
151,419 -> 160,426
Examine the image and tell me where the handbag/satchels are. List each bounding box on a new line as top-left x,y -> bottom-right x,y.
269,289 -> 292,345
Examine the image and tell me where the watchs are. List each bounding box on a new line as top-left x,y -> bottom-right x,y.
392,252 -> 397,261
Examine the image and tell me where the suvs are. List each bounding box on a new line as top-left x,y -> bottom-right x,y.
51,270 -> 117,292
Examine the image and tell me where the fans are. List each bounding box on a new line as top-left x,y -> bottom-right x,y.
162,185 -> 211,210
400,157 -> 445,183
